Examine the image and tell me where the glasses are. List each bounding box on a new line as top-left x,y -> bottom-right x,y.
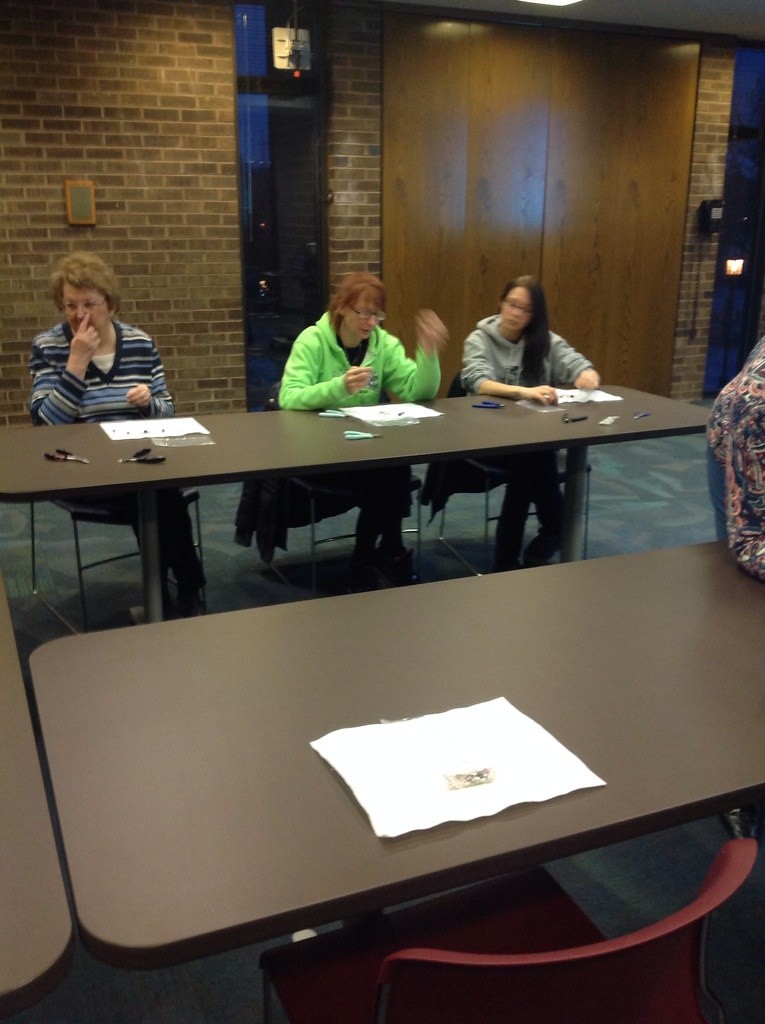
60,297 -> 106,315
502,298 -> 533,315
347,304 -> 386,321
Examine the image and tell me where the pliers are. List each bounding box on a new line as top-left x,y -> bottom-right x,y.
560,412 -> 588,423
472,400 -> 505,409
117,448 -> 167,465
44,448 -> 92,464
343,431 -> 382,440
318,409 -> 353,418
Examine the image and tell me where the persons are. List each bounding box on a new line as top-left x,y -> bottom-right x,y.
278,272 -> 451,582
705,334 -> 765,839
29,251 -> 207,616
458,272 -> 601,564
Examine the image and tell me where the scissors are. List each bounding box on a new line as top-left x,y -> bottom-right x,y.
633,410 -> 651,420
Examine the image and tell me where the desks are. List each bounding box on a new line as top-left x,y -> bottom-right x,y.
30,540 -> 765,971
0,591 -> 78,1019
1,384 -> 716,635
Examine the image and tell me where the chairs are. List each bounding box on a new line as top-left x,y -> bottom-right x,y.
437,372 -> 592,575
258,840 -> 759,1024
30,487 -> 199,636
262,382 -> 423,598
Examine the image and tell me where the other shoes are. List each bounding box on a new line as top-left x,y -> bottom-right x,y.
523,527 -> 563,563
492,561 -> 519,572
175,582 -> 205,618
373,547 -> 421,586
